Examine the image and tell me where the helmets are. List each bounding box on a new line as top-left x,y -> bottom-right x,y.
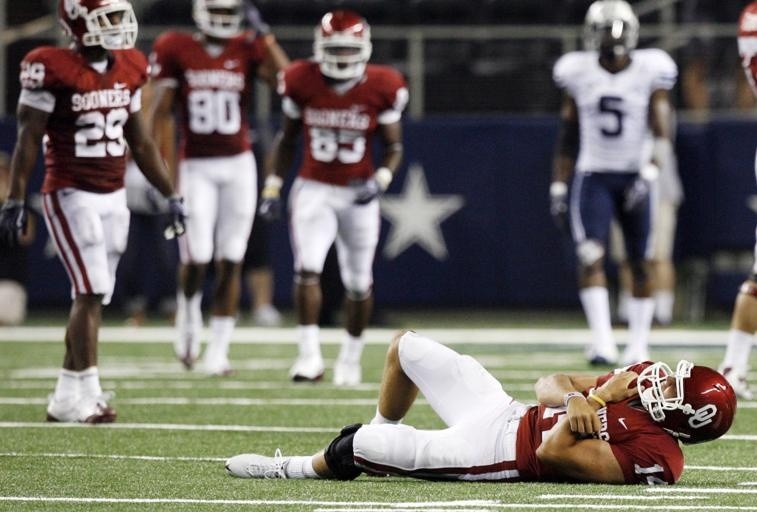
582,0 -> 640,56
637,359 -> 736,444
308,10 -> 373,81
192,0 -> 244,40
57,0 -> 139,50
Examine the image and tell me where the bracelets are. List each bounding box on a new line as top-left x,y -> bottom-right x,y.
549,181 -> 569,198
589,392 -> 606,407
261,172 -> 283,190
563,392 -> 586,407
372,167 -> 394,190
260,186 -> 280,201
637,163 -> 660,182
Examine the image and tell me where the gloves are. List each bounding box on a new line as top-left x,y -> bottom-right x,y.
550,182 -> 569,228
0,199 -> 28,245
258,174 -> 283,223
169,197 -> 186,236
624,163 -> 658,214
354,167 -> 392,204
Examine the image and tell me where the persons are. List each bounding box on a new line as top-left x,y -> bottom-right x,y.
1,1 -> 183,422
548,2 -> 678,368
142,1 -> 291,376
259,11 -> 409,388
224,329 -> 735,485
716,2 -> 757,401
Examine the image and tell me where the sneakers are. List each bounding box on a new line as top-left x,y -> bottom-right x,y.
225,448 -> 291,479
47,396 -> 73,422
717,362 -> 754,401
586,293 -> 674,365
72,390 -> 117,423
174,307 -> 361,389
